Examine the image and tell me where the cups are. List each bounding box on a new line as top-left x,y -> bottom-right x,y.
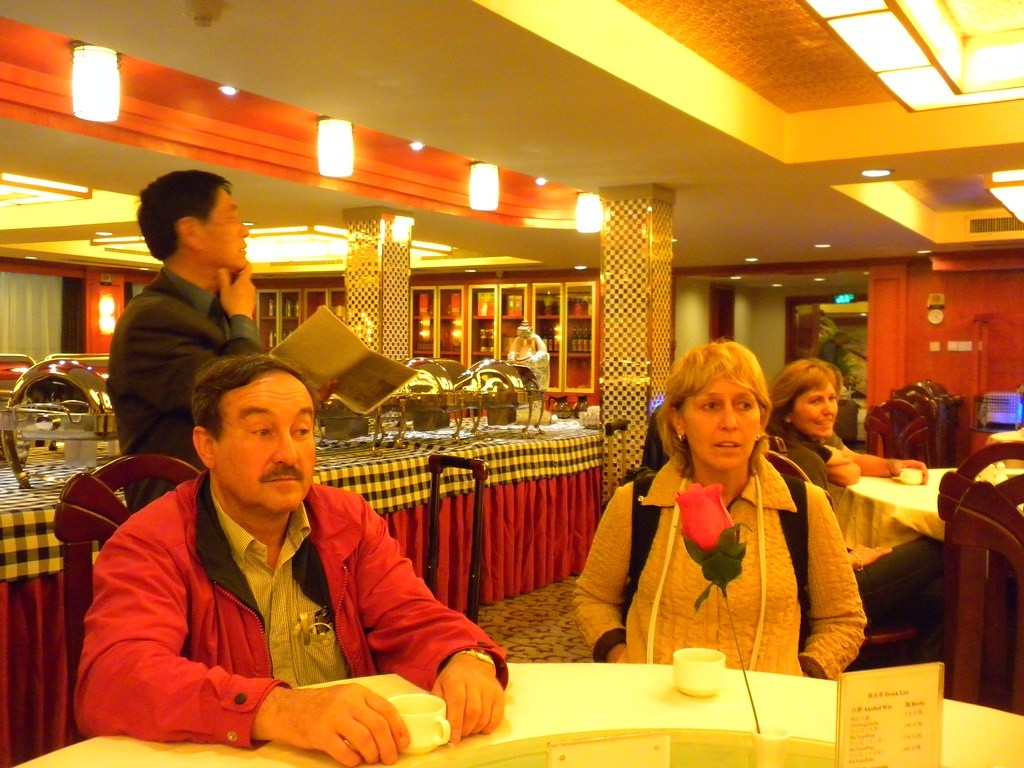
385,693 -> 449,756
899,468 -> 923,484
672,648 -> 726,698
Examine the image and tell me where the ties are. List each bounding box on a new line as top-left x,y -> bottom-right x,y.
217,306 -> 231,340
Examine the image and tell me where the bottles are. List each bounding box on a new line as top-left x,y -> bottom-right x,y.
568,295 -> 589,316
548,393 -> 601,428
571,326 -> 592,352
267,299 -> 301,349
416,331 -> 432,351
541,326 -> 561,352
479,330 -> 494,353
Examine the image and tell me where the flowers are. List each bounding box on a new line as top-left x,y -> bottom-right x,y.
675,483 -> 764,734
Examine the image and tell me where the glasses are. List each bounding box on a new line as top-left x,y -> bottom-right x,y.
314,604 -> 335,635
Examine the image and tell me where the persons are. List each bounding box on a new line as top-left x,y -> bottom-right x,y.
105,169 -> 338,511
572,338 -> 868,680
818,316 -> 867,398
768,358 -> 944,648
74,355 -> 509,768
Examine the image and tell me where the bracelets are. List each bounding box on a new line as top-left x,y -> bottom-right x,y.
454,650 -> 494,665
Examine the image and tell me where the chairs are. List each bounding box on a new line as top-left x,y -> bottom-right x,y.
865,381 -> 949,470
938,440 -> 1024,710
53,456 -> 201,741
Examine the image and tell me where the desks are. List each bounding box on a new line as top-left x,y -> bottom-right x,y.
1,424 -> 604,764
17,660 -> 1024,768
844,468 -> 946,541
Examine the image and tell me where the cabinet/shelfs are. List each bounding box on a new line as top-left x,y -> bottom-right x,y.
255,287 -> 345,349
409,284 -> 599,404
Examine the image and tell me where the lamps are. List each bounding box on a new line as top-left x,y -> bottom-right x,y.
71,40 -> 121,122
316,115 -> 354,177
469,161 -> 500,211
